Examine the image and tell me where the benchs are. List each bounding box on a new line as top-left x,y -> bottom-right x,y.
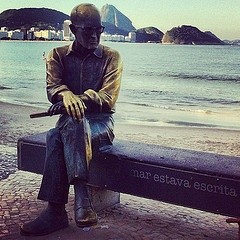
17,132 -> 240,219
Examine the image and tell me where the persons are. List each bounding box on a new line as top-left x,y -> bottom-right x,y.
20,3 -> 122,236
